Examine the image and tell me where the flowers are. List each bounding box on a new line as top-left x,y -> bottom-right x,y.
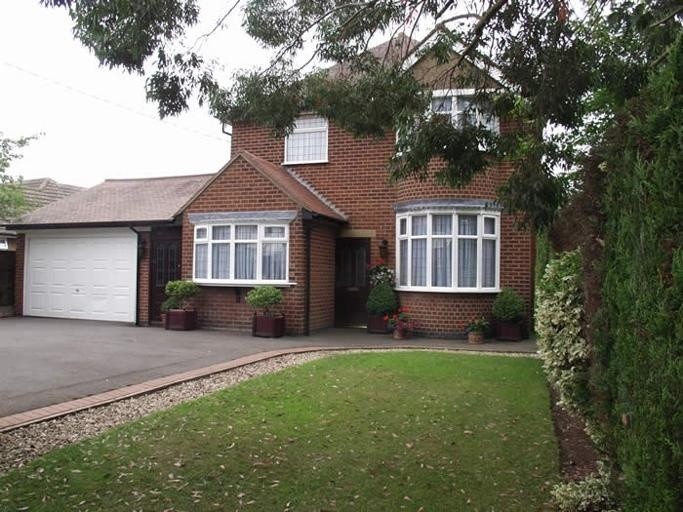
384,306 -> 419,330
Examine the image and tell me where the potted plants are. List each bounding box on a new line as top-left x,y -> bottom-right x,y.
245,285 -> 286,337
366,282 -> 400,333
160,280 -> 202,329
463,283 -> 530,344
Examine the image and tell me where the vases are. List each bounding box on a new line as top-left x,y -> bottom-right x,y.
392,329 -> 409,339
